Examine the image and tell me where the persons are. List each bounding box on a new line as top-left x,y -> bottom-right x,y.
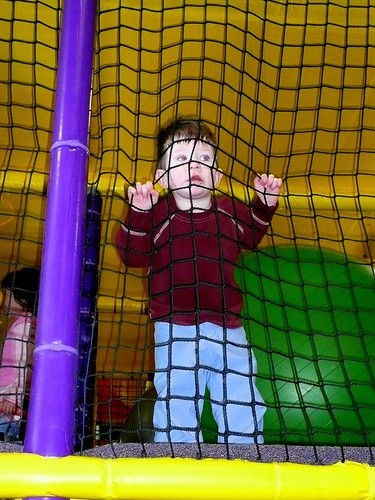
116,117 -> 282,444
0,267 -> 40,440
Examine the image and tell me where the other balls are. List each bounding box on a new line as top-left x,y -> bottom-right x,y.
125,397 -> 155,444
202,245 -> 374,446
